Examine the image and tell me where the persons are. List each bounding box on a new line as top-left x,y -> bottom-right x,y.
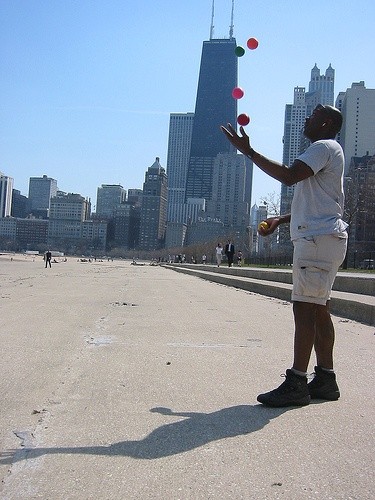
214,243 -> 224,267
238,256 -> 242,267
219,102 -> 352,412
224,240 -> 235,267
44,249 -> 52,268
202,253 -> 207,265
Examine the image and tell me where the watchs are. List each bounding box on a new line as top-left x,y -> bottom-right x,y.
246,149 -> 256,159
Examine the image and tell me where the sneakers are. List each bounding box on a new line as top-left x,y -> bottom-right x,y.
306,366 -> 341,400
258,368 -> 312,408
